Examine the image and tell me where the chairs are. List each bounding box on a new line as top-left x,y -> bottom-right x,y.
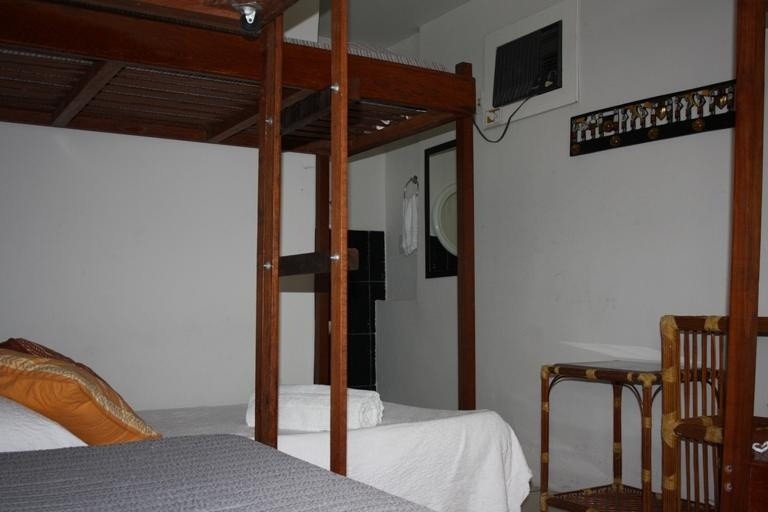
660,315 -> 768,511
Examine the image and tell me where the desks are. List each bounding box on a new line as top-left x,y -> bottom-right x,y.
540,358 -> 719,512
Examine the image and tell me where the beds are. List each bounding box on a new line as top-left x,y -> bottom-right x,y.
1,276 -> 534,512
1,1 -> 476,276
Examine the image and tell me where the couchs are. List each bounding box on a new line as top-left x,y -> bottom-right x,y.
0,433 -> 429,512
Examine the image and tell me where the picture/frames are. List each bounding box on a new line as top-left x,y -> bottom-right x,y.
424,139 -> 457,278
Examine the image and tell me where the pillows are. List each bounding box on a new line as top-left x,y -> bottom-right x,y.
0,336 -> 161,446
1,393 -> 88,452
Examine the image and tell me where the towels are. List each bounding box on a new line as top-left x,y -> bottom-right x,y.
398,195 -> 418,254
246,384 -> 385,432
